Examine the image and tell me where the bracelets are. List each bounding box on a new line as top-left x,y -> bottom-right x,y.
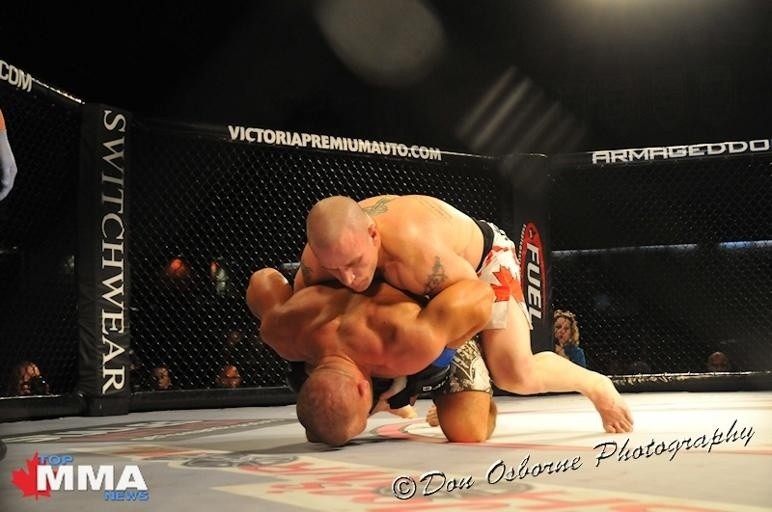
432,348 -> 457,369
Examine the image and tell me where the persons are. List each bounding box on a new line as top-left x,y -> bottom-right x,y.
707,352 -> 732,372
4,360 -> 49,398
553,310 -> 586,366
246,267 -> 496,446
293,194 -> 633,434
147,257 -> 247,390
